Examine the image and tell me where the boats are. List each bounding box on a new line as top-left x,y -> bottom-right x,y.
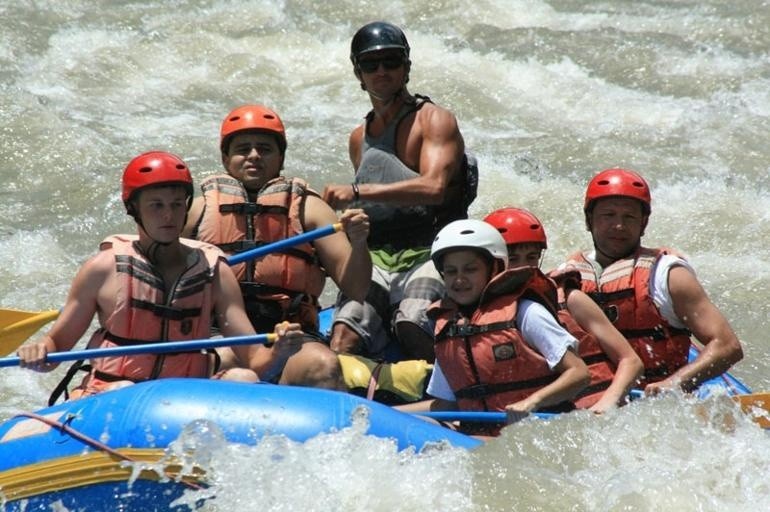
0,302 -> 769,511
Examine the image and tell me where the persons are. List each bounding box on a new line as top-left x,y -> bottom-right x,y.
483,208 -> 646,416
322,21 -> 468,362
183,105 -> 373,394
390,218 -> 592,425
16,151 -> 304,403
547,169 -> 744,399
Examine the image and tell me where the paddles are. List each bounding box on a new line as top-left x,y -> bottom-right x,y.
0,222 -> 343,359
413,393 -> 769,431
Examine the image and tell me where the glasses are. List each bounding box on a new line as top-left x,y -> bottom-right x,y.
358,50 -> 405,73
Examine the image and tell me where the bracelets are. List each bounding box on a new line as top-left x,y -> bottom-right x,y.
351,182 -> 361,201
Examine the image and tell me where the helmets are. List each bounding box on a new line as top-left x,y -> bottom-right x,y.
483,207 -> 547,250
123,151 -> 193,216
583,169 -> 651,216
429,218 -> 509,274
220,103 -> 287,151
351,21 -> 410,60
